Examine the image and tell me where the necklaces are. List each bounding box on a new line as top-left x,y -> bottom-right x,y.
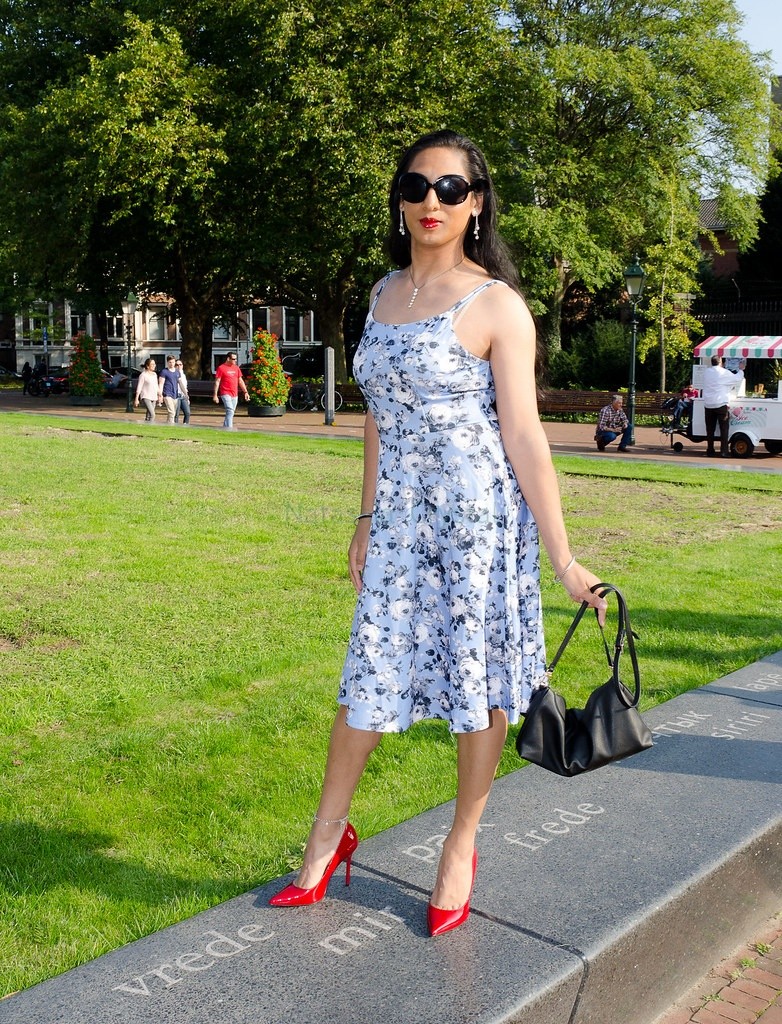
409,257 -> 467,309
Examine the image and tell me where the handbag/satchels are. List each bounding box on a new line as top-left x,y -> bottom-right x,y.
516,582 -> 653,777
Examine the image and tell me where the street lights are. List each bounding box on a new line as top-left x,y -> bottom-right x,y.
278,335 -> 285,365
620,250 -> 647,445
120,289 -> 138,413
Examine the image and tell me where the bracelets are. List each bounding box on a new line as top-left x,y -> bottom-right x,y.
354,513 -> 373,526
553,558 -> 576,584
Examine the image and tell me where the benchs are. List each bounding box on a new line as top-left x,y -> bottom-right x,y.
539,389 -> 690,423
109,378 -> 138,398
188,380 -> 249,403
293,385 -> 368,414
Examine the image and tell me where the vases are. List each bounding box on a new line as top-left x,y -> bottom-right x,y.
247,405 -> 288,417
68,396 -> 104,406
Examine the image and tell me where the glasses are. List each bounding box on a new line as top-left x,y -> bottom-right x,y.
175,363 -> 183,367
396,171 -> 475,205
230,358 -> 238,361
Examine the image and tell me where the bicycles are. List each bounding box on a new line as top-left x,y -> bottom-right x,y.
288,376 -> 343,412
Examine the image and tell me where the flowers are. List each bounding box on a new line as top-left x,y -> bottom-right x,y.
67,333 -> 107,396
247,325 -> 293,407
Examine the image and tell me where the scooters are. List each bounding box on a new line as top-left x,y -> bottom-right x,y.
33,369 -> 51,397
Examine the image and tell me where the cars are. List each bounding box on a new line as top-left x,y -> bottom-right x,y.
108,366 -> 142,388
65,368 -> 115,399
0,366 -> 22,377
27,367 -> 70,395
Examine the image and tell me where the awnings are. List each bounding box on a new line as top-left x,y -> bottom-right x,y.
694,336 -> 782,359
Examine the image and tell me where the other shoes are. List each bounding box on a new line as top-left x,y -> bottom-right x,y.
617,446 -> 630,453
664,426 -> 678,433
597,441 -> 605,452
707,448 -> 718,457
721,447 -> 733,457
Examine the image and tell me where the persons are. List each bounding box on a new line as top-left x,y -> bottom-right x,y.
134,355 -> 190,424
213,353 -> 250,427
267,127 -> 608,935
701,356 -> 746,456
666,383 -> 698,427
594,395 -> 634,452
104,369 -> 128,390
22,359 -> 47,395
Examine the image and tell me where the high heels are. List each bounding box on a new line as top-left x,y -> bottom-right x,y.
428,846 -> 478,936
269,821 -> 358,907
